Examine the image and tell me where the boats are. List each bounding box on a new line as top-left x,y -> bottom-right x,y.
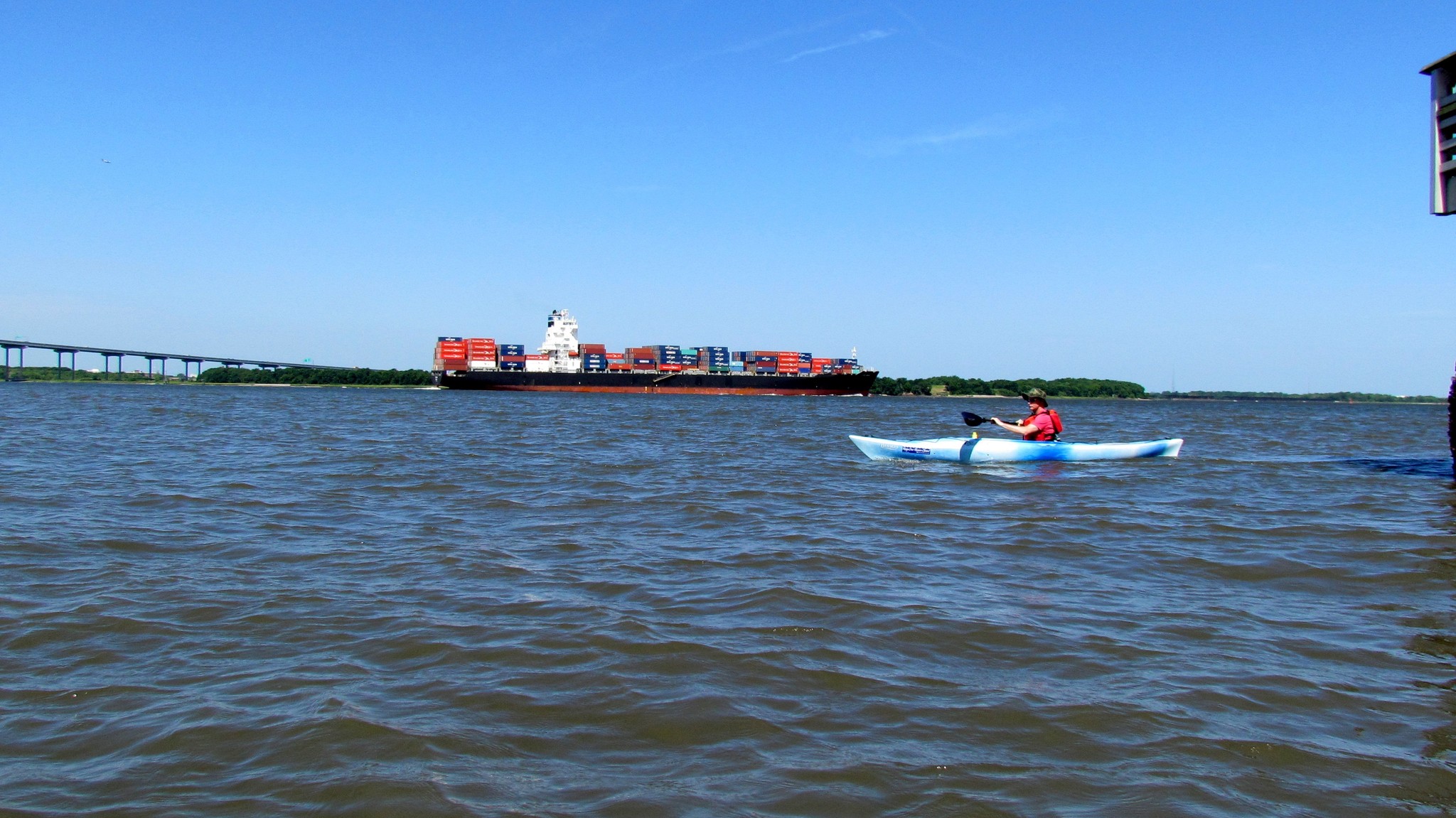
847,433 -> 1184,464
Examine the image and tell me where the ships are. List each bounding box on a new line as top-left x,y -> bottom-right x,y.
426,308 -> 881,396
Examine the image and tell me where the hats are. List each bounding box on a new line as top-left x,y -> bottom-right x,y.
1021,388 -> 1048,408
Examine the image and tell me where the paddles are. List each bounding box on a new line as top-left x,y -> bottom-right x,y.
961,411 -> 1019,428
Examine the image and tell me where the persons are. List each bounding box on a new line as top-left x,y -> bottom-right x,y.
990,387 -> 1063,441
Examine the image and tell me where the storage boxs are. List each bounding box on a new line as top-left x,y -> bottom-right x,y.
433,336 -> 550,370
578,343 -> 859,377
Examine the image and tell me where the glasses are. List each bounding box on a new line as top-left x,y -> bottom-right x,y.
1026,398 -> 1036,403
